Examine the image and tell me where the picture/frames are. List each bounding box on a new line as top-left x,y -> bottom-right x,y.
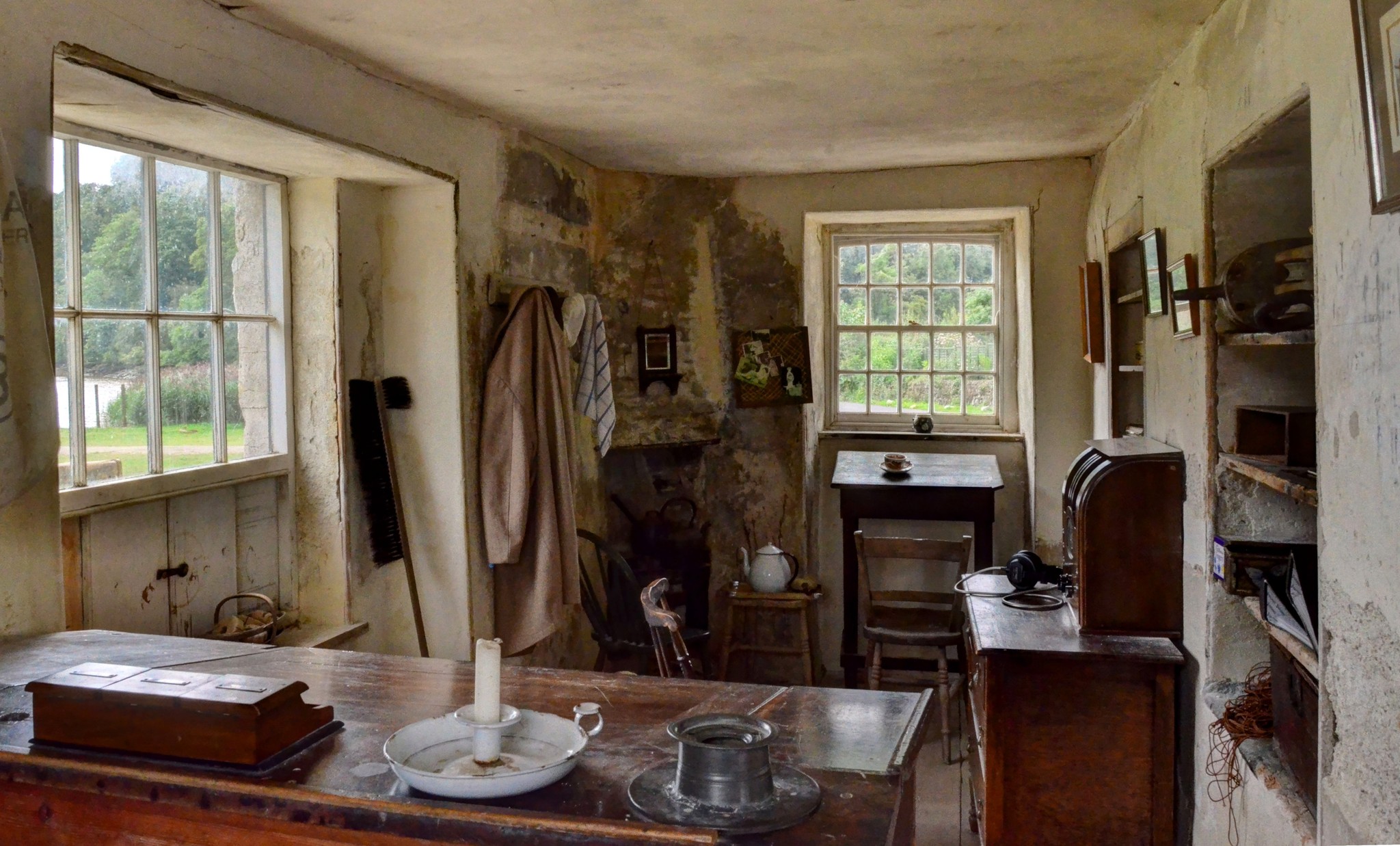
1165,252 -> 1196,340
1137,227 -> 1168,317
636,324 -> 678,394
1348,0 -> 1400,216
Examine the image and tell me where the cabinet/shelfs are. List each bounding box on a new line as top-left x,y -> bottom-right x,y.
956,571 -> 1186,846
1214,325 -> 1317,681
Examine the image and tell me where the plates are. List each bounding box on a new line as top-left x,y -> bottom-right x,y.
879,462 -> 914,472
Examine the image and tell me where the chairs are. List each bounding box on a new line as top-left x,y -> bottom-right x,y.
574,528 -> 710,677
640,577 -> 694,678
853,530 -> 974,763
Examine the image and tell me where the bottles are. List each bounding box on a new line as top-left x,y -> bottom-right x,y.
913,414 -> 934,434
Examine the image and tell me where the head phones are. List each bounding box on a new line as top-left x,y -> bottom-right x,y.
1007,550 -> 1064,591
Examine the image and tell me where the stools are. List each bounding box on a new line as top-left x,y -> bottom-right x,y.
719,582 -> 814,686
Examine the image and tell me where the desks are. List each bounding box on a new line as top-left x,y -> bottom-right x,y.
830,448 -> 1005,695
0,627 -> 933,846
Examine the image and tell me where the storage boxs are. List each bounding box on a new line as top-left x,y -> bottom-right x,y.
1213,541 -> 1291,595
1266,635 -> 1318,811
1232,403 -> 1317,467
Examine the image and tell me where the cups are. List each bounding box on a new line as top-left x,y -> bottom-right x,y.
884,454 -> 906,470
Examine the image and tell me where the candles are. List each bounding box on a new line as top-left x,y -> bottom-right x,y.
474,637 -> 501,724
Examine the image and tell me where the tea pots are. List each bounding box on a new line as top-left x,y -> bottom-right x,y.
659,496 -> 710,568
741,542 -> 798,592
